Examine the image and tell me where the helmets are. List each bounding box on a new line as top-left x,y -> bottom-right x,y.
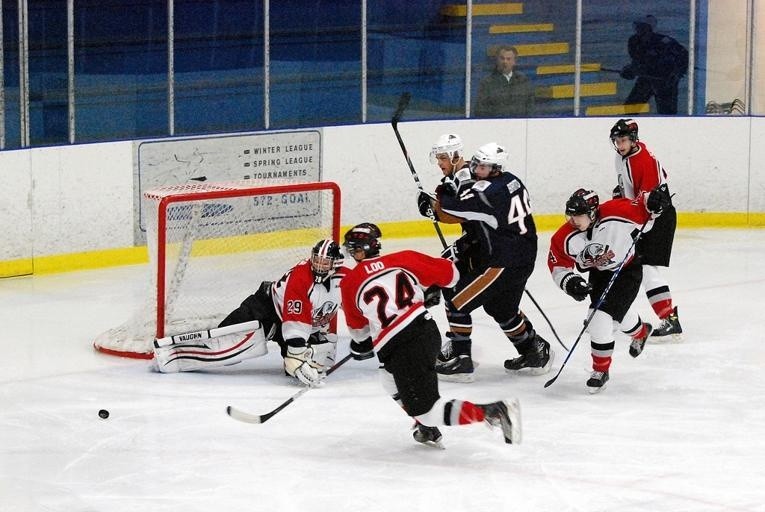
632,15 -> 656,33
428,133 -> 464,166
610,120 -> 637,156
309,238 -> 341,282
468,144 -> 507,181
564,188 -> 599,231
342,223 -> 382,262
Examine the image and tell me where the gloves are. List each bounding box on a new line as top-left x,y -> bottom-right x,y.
348,336 -> 374,361
417,192 -> 438,224
646,189 -> 667,213
424,285 -> 440,309
284,345 -> 335,386
570,281 -> 593,300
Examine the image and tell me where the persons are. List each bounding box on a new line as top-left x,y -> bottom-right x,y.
474,45 -> 536,117
546,188 -> 663,389
609,118 -> 683,337
619,15 -> 689,115
435,142 -> 549,375
339,223 -> 513,445
149,238 -> 353,388
415,134 -> 551,367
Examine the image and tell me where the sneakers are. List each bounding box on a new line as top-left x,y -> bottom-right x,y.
434,306 -> 684,388
478,402 -> 512,444
411,424 -> 442,444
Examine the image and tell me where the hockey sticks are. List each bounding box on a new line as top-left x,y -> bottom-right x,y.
226,354 -> 354,424
542,213 -> 654,387
390,90 -> 449,250
